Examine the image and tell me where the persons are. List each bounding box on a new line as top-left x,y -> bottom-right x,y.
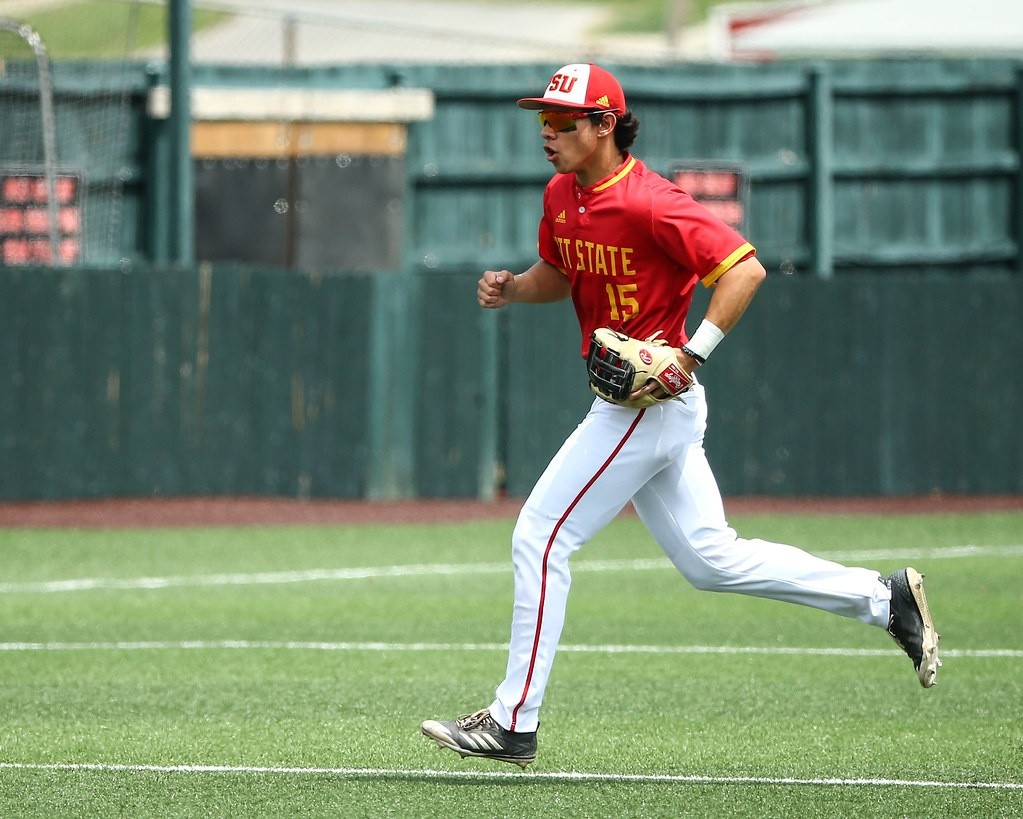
422,62 -> 941,770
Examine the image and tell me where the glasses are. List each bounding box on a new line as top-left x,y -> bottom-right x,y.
537,108 -> 622,133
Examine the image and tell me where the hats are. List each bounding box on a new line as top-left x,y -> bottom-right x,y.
516,62 -> 625,119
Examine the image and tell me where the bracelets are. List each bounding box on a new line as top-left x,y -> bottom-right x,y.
680,345 -> 706,364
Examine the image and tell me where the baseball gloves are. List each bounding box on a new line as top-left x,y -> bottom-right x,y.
584,326 -> 697,411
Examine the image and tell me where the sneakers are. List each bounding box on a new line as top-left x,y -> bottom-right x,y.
878,567 -> 943,688
420,709 -> 540,769
